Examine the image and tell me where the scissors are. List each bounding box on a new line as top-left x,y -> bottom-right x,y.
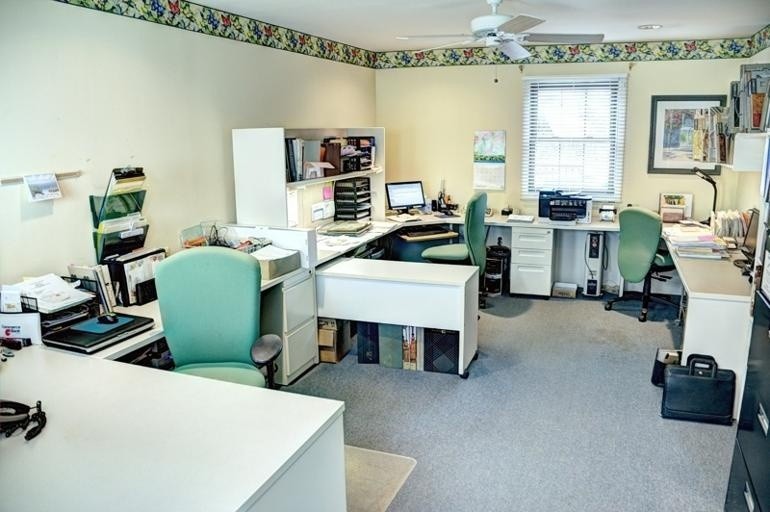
438,192 -> 445,200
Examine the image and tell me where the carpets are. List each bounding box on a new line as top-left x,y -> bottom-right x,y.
345,444 -> 417,511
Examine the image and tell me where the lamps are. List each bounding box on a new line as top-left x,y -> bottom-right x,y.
393,1 -> 604,62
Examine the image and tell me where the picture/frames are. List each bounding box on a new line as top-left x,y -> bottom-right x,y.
648,95 -> 727,174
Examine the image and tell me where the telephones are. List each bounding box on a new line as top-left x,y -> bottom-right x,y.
0,399 -> 30,430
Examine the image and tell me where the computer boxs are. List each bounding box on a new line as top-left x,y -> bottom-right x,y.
483,257 -> 504,296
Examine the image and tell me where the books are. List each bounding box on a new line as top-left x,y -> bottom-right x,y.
658,191 -> 694,226
41,311 -> 89,330
14,272 -> 94,314
710,209 -> 752,239
97,162 -> 167,309
249,244 -> 301,280
707,62 -> 770,164
68,262 -> 117,313
317,219 -> 374,237
665,226 -> 727,260
285,138 -> 305,183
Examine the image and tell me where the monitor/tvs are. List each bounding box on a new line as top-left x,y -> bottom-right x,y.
733,207 -> 760,268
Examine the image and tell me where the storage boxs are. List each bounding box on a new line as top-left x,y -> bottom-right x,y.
317,318 -> 352,363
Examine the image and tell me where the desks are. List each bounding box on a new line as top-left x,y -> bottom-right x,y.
0,225 -> 346,512
661,220 -> 754,421
317,211 -> 624,378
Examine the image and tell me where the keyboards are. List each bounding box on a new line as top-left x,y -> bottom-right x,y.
402,225 -> 449,237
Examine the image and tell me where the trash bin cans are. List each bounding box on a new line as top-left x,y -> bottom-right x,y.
485,245 -> 511,293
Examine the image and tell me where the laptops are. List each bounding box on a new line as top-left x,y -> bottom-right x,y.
385,181 -> 425,215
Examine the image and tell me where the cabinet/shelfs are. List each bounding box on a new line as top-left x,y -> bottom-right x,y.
232,127 -> 386,228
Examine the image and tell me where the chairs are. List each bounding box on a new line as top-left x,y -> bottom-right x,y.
420,192 -> 487,308
605,206 -> 679,322
155,246 -> 284,389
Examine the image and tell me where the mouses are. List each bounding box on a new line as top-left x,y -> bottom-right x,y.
97,316 -> 118,324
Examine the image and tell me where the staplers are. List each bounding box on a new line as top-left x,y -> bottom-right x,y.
0,337 -> 32,350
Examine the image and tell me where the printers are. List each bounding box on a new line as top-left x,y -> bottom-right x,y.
538,190 -> 593,225
